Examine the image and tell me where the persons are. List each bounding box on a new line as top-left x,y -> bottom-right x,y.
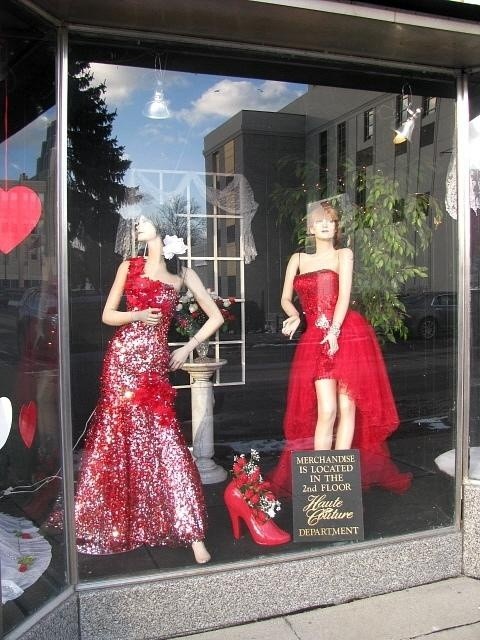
41,204 -> 224,565
269,205 -> 414,496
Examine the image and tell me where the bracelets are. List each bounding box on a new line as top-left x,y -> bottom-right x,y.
328,324 -> 340,337
192,336 -> 200,345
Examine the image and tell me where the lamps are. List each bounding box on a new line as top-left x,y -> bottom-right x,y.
390,84 -> 425,144
142,52 -> 173,120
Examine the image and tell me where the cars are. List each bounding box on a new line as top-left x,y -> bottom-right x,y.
15,280 -> 104,364
389,288 -> 459,343
0,284 -> 30,308
221,297 -> 268,337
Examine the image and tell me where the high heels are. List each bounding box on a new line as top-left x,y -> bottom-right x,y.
224,479 -> 292,546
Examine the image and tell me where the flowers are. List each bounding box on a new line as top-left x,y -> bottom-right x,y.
229,448 -> 282,525
3,524 -> 32,540
16,537 -> 36,575
162,235 -> 189,261
175,290 -> 236,340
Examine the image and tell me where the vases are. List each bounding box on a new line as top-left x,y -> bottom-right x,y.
194,338 -> 209,363
